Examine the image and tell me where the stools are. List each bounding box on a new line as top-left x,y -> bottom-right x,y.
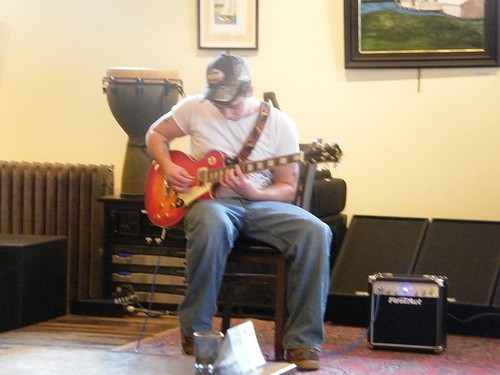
0,234 -> 68,332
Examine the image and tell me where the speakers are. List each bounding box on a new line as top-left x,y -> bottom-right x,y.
328,216 -> 500,354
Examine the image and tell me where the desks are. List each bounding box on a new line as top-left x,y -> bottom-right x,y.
1,341 -> 298,375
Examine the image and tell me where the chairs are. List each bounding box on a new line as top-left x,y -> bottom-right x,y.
219,161 -> 316,361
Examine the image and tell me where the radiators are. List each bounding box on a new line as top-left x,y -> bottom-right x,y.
0,162 -> 114,300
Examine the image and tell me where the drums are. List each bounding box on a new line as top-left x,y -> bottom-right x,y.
100,64 -> 184,199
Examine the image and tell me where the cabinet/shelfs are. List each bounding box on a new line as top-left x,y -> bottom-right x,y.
97,193 -> 187,306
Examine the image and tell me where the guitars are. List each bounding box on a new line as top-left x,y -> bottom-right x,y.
143,138 -> 344,229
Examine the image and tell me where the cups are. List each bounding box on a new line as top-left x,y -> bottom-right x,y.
193,331 -> 223,375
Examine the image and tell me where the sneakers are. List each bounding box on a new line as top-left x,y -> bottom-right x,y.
287,347 -> 319,369
180,327 -> 195,356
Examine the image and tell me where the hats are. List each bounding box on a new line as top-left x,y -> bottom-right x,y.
204,52 -> 251,102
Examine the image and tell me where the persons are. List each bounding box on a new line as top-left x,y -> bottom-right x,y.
147,52 -> 334,372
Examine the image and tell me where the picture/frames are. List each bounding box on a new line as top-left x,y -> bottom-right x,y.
344,0 -> 500,69
198,0 -> 258,50
